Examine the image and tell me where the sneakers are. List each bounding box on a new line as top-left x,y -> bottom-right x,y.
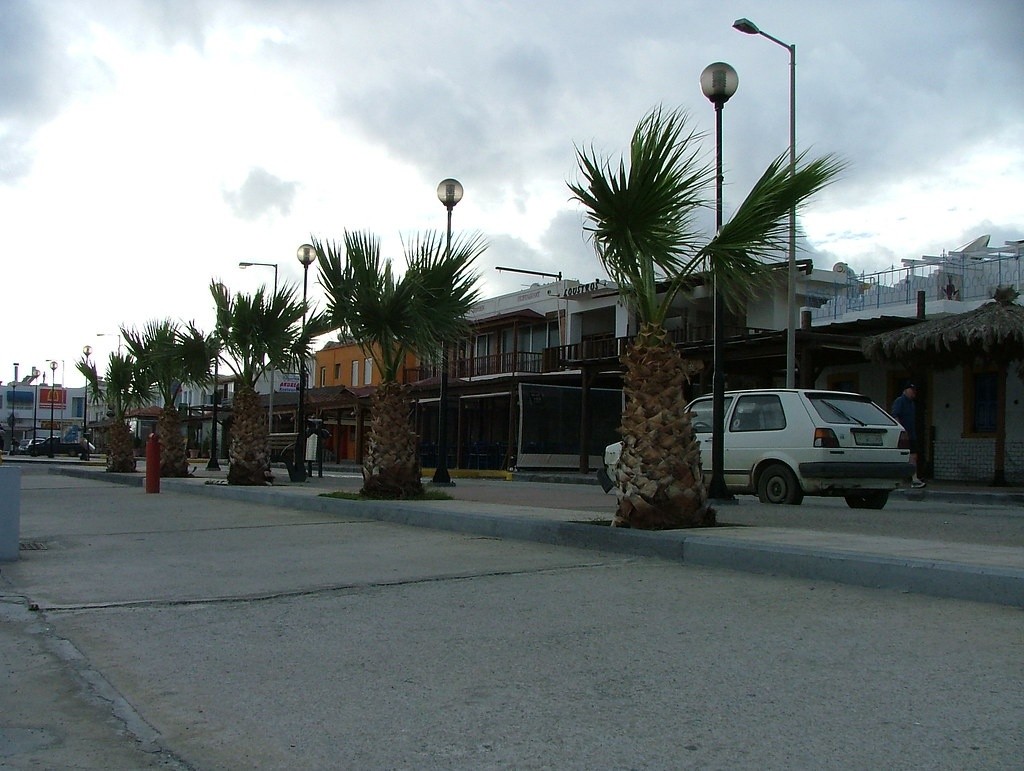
893,483 -> 906,492
911,481 -> 926,488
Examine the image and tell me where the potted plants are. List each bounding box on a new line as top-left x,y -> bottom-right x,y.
131,437 -> 143,457
206,429 -> 219,459
189,430 -> 200,459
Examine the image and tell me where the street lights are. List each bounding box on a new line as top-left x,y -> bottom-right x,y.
80,345 -> 94,461
732,17 -> 797,389
46,359 -> 65,444
48,361 -> 59,458
428,179 -> 464,487
239,260 -> 279,435
10,380 -> 19,454
32,370 -> 41,456
289,243 -> 316,478
700,60 -> 740,504
96,333 -> 122,415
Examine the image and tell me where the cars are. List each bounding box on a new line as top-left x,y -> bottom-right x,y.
18,438 -> 86,456
596,384 -> 918,511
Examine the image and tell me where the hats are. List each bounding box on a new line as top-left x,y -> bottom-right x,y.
904,381 -> 920,390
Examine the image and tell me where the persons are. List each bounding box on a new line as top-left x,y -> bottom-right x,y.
892,385 -> 926,489
